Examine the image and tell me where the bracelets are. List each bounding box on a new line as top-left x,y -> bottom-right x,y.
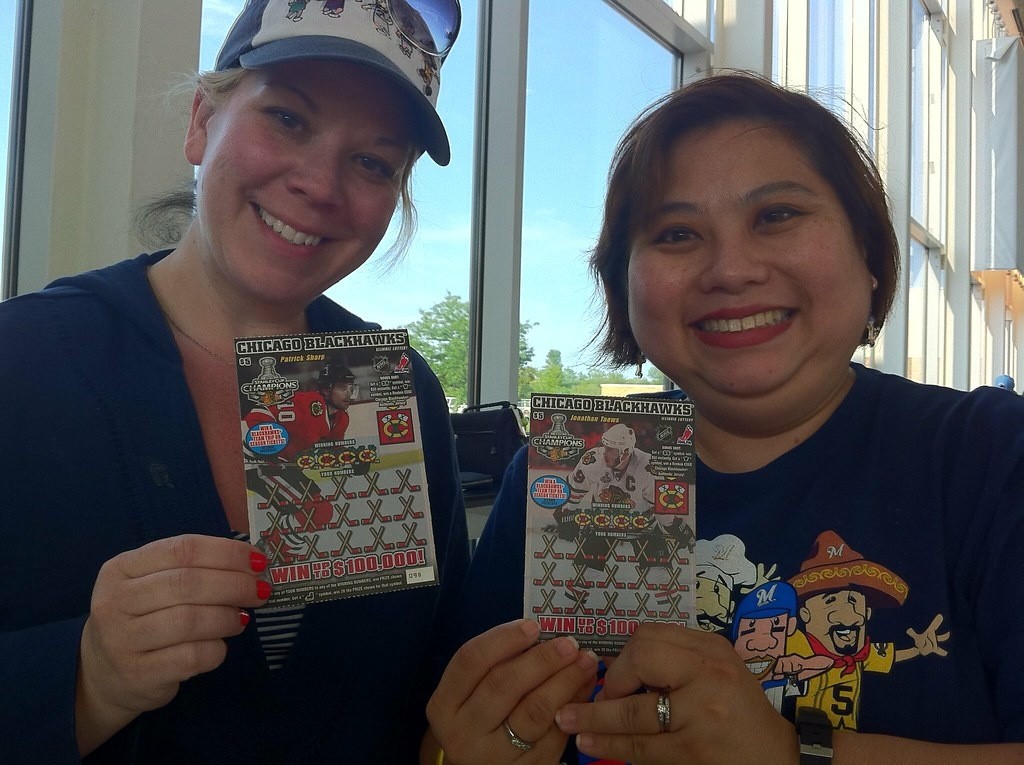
795,706 -> 834,765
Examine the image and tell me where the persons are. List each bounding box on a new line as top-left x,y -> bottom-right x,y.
0,0 -> 472,765
554,423 -> 695,612
241,364 -> 370,591
994,375 -> 1018,394
425,74 -> 1024,765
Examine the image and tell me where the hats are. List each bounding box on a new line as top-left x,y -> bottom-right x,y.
215,0 -> 454,168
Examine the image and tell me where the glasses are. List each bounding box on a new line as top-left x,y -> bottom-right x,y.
388,0 -> 463,67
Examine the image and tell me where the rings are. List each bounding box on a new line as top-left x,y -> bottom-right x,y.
657,689 -> 671,733
503,720 -> 533,752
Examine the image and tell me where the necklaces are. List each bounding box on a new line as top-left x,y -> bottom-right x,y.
161,306 -> 236,370
